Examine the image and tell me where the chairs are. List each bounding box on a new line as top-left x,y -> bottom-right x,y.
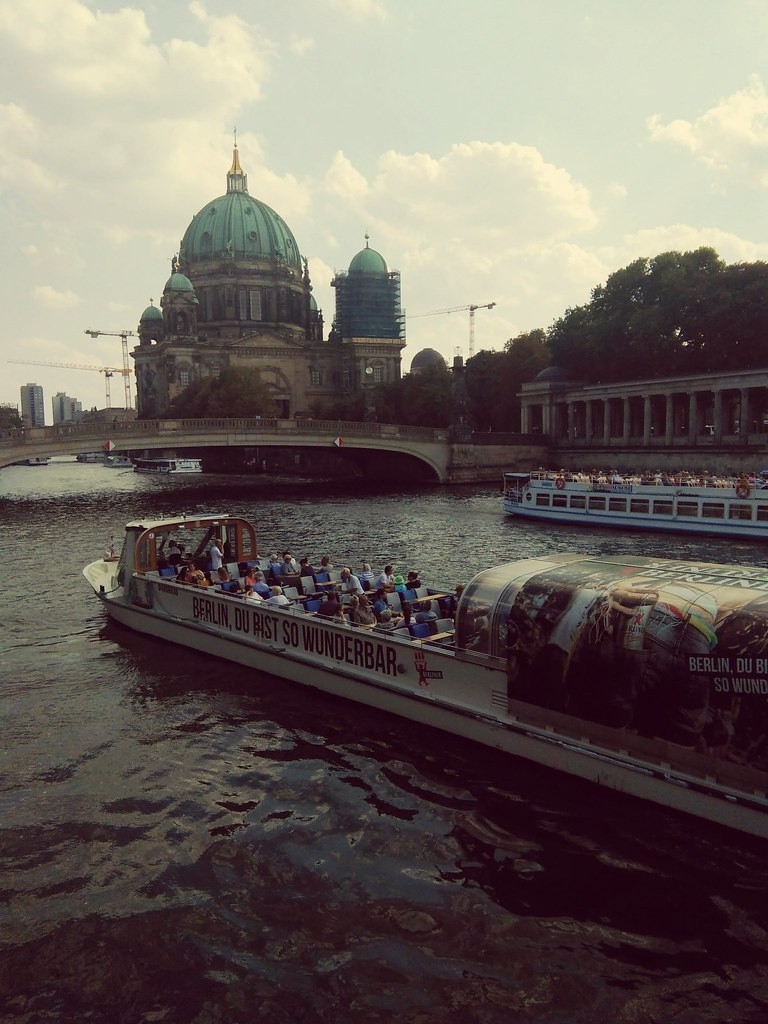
545,472 -> 737,489
145,559 -> 458,647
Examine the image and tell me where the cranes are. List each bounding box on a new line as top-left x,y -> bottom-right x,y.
406,300 -> 496,359
7,329 -> 136,409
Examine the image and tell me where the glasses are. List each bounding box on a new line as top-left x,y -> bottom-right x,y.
250,570 -> 254,572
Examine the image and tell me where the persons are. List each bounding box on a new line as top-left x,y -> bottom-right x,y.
539,463 -> 768,490
176,539 -> 491,654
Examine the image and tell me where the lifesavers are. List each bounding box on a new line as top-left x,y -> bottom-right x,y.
556,478 -> 565,490
736,484 -> 750,499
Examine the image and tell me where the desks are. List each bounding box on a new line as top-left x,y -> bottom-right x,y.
413,632 -> 454,644
410,593 -> 452,613
358,617 -> 404,630
305,604 -> 353,617
314,580 -> 342,590
453,606 -> 492,634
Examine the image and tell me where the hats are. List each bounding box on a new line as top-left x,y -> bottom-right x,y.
379,609 -> 392,617
270,554 -> 277,559
270,586 -> 282,592
341,568 -> 350,574
393,575 -> 405,584
284,555 -> 291,559
415,613 -> 426,623
454,584 -> 464,591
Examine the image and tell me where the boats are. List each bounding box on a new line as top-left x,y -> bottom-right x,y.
500,471 -> 768,542
82,512 -> 768,844
72,452 -> 204,475
13,456 -> 53,466
